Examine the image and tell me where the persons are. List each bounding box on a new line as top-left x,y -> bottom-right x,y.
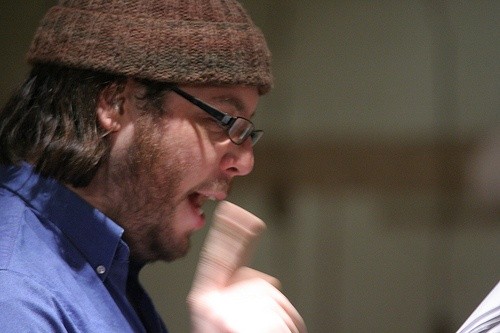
0,1 -> 305,333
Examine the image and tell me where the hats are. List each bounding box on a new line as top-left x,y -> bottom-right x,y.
25,2 -> 276,92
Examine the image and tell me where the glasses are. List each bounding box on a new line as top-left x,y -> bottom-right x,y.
170,87 -> 265,148
